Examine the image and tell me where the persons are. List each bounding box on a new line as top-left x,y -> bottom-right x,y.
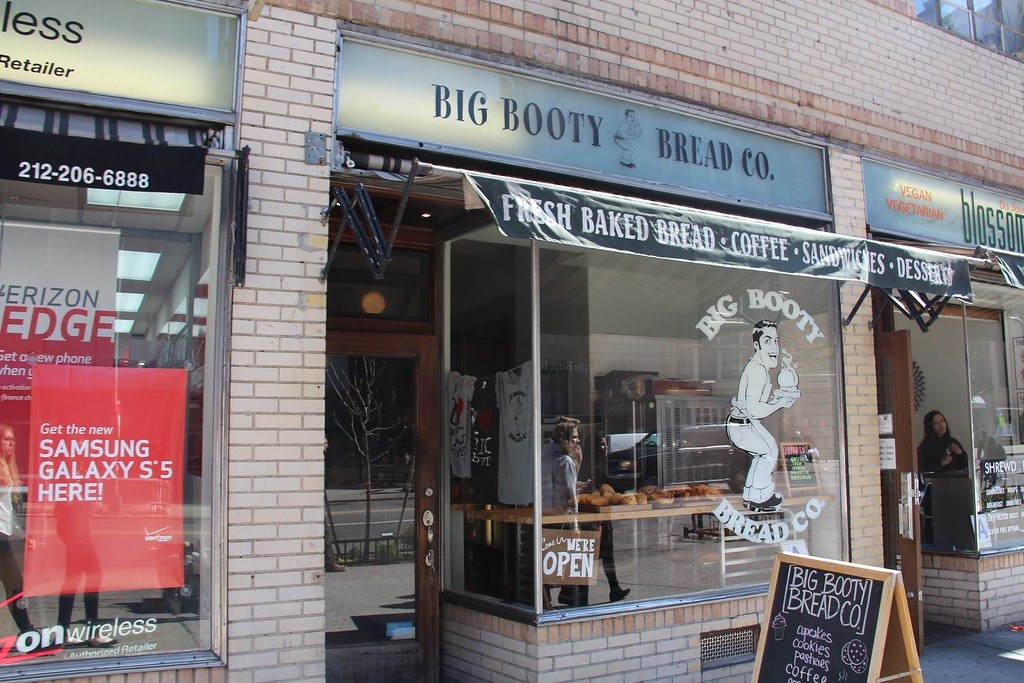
540,417 -> 583,605
557,432 -> 630,605
0,425 -> 58,655
912,410 -> 975,550
323,436 -> 348,573
976,430 -> 1006,486
54,439 -> 118,647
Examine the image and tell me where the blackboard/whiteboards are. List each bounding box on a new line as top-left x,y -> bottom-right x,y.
750,554 -> 897,683
779,441 -> 821,491
981,458 -> 1024,547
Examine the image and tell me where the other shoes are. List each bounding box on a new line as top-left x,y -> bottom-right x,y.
54,638 -> 89,647
86,637 -> 120,647
558,591 -> 574,607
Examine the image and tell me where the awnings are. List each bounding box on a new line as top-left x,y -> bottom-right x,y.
355,153 -> 991,333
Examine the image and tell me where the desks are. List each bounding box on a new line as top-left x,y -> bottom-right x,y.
465,492 -> 835,608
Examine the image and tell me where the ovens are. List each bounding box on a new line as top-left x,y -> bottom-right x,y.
594,395 -> 746,493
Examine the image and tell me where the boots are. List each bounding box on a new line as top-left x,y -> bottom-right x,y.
606,572 -> 630,602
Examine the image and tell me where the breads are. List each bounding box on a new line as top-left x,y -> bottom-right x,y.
567,484 -> 723,506
